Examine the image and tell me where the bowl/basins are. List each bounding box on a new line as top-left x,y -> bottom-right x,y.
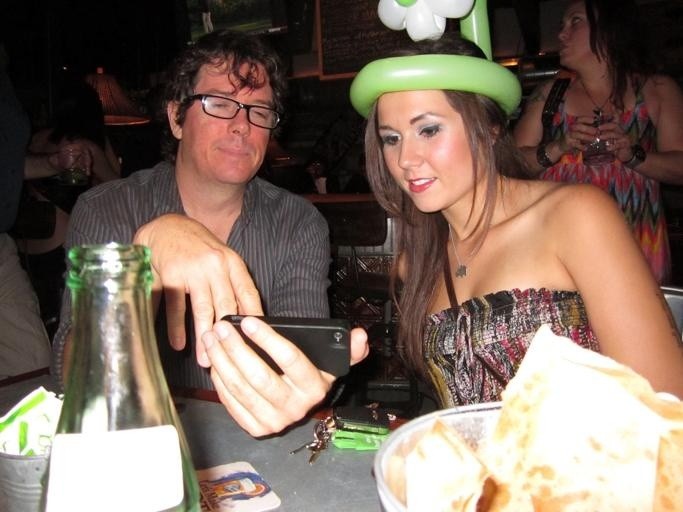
373,403 -> 532,512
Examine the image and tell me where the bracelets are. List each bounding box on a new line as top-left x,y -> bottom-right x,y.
536,142 -> 555,169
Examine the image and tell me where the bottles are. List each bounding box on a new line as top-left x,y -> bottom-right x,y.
40,242 -> 201,512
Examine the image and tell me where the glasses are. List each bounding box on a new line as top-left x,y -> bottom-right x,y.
183,94 -> 284,131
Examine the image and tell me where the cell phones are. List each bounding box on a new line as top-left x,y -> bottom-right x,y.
215,312 -> 351,379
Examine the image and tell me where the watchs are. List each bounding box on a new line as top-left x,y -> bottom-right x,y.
621,143 -> 646,171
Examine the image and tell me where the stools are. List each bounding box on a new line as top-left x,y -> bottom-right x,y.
353,321 -> 421,422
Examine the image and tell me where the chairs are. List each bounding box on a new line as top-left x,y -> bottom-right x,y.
659,283 -> 683,338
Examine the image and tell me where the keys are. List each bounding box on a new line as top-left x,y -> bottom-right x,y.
291,420 -> 330,465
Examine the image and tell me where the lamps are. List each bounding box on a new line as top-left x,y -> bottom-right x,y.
76,68 -> 151,128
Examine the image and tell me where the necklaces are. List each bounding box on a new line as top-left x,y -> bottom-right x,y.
577,76 -> 617,117
447,222 -> 491,278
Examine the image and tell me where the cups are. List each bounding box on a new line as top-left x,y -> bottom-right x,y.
0,453 -> 44,512
579,119 -> 618,164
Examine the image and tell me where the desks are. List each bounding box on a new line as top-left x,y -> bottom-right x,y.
0,370 -> 407,510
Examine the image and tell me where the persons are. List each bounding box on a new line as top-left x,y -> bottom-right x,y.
511,0 -> 683,296
50,28 -> 370,439
348,36 -> 683,412
3,39 -> 118,382
